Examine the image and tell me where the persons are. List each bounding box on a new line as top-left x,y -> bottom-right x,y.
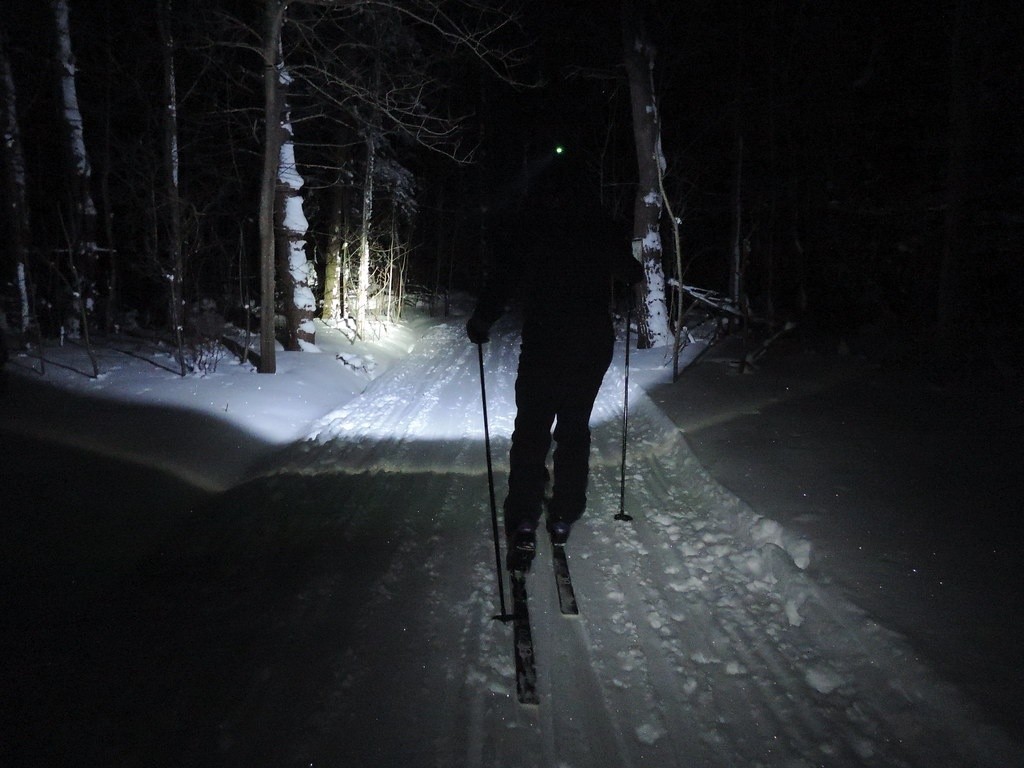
466,156 -> 643,571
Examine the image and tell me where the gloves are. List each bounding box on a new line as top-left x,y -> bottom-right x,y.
466,305 -> 492,344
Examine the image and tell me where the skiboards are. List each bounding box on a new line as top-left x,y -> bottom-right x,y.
510,533 -> 583,706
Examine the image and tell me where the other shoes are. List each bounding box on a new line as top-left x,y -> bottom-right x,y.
547,517 -> 570,547
511,521 -> 536,559
